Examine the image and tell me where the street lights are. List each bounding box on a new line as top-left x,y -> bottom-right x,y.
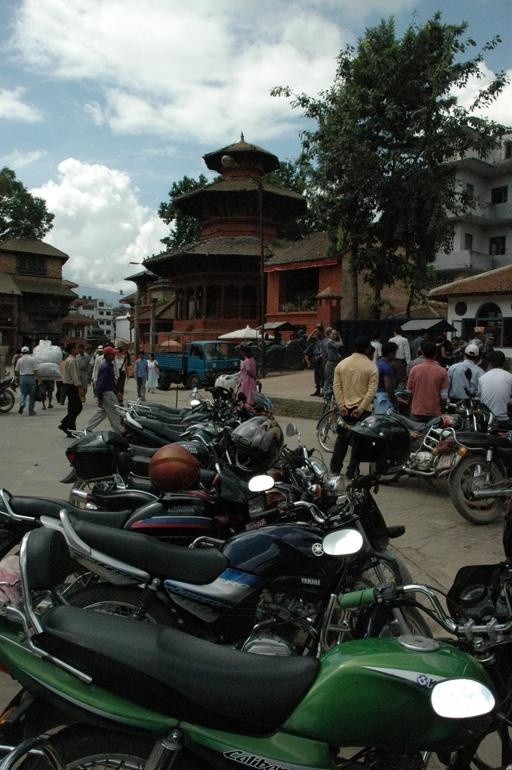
219,155 -> 266,379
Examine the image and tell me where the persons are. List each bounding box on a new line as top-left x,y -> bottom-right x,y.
286,335 -> 295,349
296,329 -> 313,369
307,322 -> 512,480
238,344 -> 259,410
11,338 -> 161,439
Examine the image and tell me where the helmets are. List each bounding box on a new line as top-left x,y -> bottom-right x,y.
150,445 -> 200,492
231,417 -> 284,474
215,375 -> 237,400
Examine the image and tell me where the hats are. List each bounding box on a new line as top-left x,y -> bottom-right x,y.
22,347 -> 29,352
465,344 -> 479,356
104,347 -> 119,353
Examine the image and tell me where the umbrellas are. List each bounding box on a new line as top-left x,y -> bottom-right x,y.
159,338 -> 183,348
217,325 -> 276,342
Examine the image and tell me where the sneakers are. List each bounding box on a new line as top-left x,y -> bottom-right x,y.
59,423 -> 77,437
18,406 -> 24,414
310,390 -> 320,396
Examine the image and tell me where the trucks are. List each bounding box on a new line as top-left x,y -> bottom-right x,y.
140,340 -> 241,390
